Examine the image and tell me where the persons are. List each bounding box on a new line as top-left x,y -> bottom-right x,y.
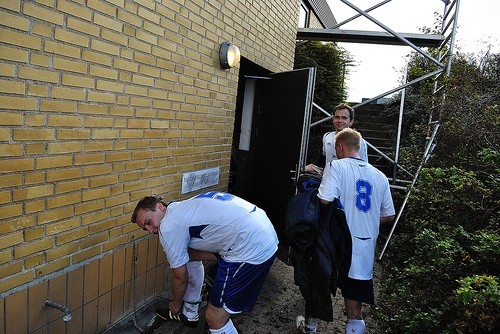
295,105 -> 395,334
131,191 -> 280,334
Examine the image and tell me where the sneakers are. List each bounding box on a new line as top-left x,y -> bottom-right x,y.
295,315 -> 316,334
155,308 -> 199,328
